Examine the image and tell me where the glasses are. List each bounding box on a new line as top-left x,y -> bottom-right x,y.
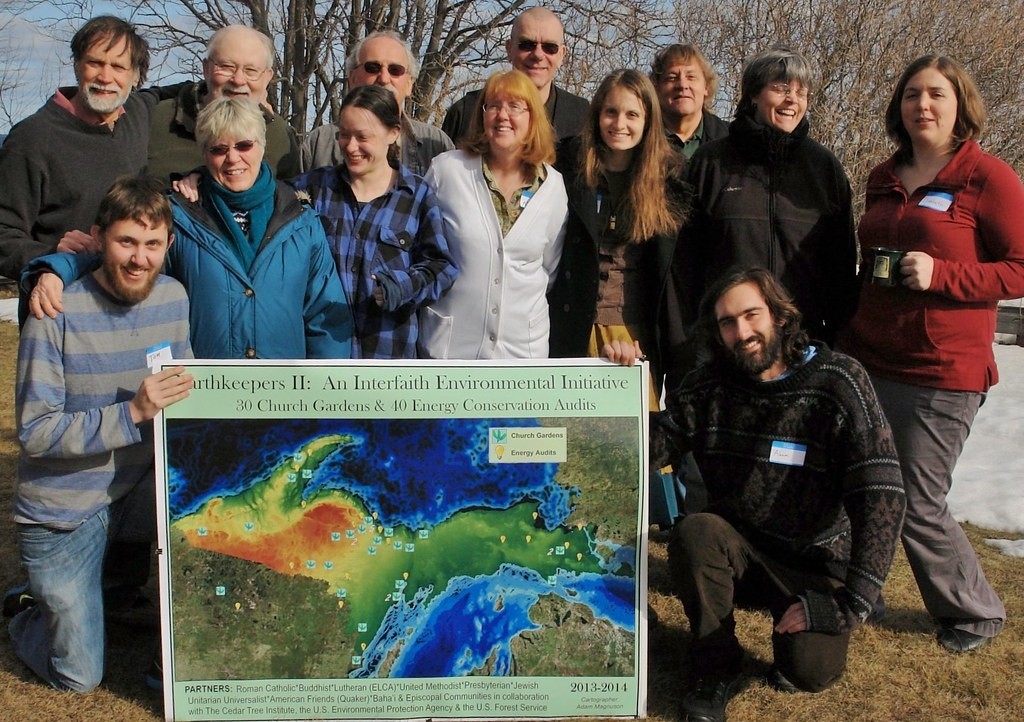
208,56 -> 267,81
352,61 -> 408,76
205,138 -> 256,155
765,83 -> 808,98
482,102 -> 530,116
511,39 -> 564,55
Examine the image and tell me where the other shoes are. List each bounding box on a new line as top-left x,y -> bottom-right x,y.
101,582 -> 157,625
866,593 -> 885,626
937,628 -> 989,654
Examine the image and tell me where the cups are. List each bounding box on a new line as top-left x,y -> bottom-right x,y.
868,246 -> 906,288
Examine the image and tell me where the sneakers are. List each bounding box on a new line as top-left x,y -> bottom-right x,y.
148,654 -> 162,691
681,665 -> 741,722
766,661 -> 801,693
3,580 -> 37,617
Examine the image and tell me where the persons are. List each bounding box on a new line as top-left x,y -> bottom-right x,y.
441,6 -> 592,357
17,93 -> 353,359
147,25 -> 297,185
0,15 -> 274,283
3,174 -> 195,693
649,41 -> 730,163
296,28 -> 454,181
679,49 -> 856,369
417,69 -> 568,359
172,82 -> 457,359
832,53 -> 1024,651
601,265 -> 906,722
545,70 -> 692,529
